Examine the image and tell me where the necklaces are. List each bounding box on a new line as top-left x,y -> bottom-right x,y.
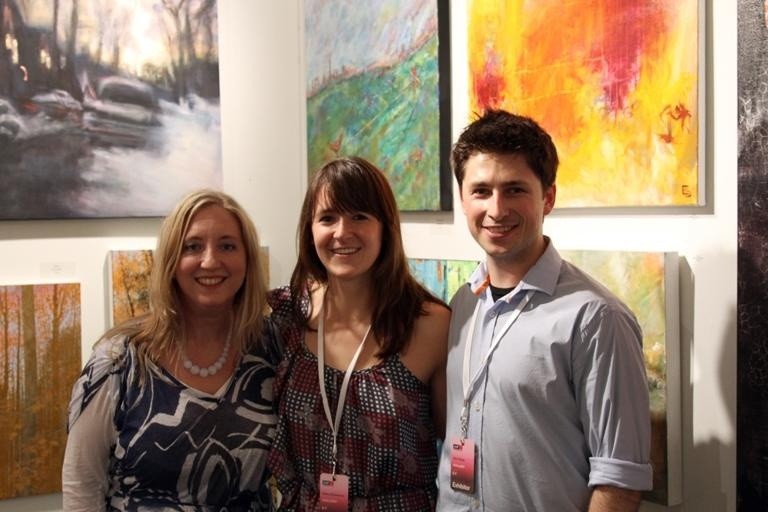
170,308 -> 236,380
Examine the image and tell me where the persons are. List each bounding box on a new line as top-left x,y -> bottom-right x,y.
61,182 -> 285,511
255,152 -> 459,511
430,107 -> 657,511
